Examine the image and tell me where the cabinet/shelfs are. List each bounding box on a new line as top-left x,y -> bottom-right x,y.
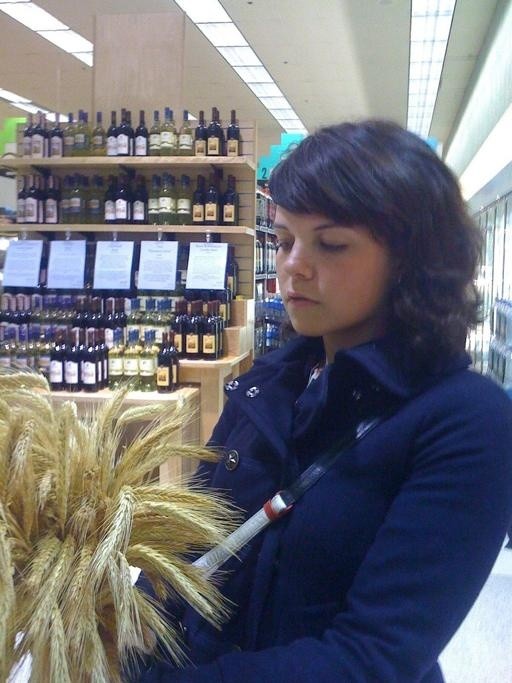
253,188 -> 295,358
1,122 -> 260,491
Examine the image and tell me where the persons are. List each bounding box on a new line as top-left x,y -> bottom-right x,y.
120,118 -> 512,681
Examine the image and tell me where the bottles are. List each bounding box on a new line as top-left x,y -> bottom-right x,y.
256,194 -> 288,351
1,244 -> 238,394
22,105 -> 240,158
487,297 -> 511,389
15,170 -> 240,226
